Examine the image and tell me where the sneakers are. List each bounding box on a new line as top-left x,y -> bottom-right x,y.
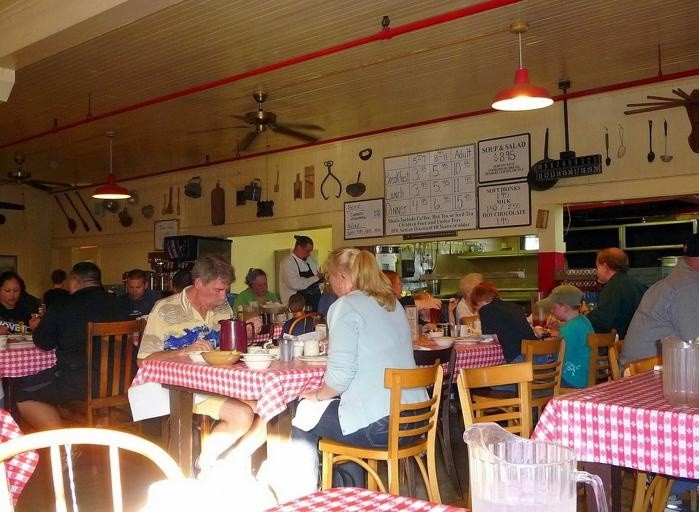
646,483 -> 686,512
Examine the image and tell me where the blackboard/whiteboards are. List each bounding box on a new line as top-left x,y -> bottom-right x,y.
383,143 -> 479,235
344,197 -> 384,241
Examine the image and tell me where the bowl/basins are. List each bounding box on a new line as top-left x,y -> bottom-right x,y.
184,349 -> 242,366
431,336 -> 454,347
238,353 -> 274,370
428,330 -> 444,339
358,148 -> 372,161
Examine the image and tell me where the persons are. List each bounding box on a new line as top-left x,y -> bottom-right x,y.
2,262 -> 190,471
443,236 -> 699,511
137,235 -> 442,491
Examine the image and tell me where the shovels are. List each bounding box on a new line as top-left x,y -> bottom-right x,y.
273,171 -> 279,192
162,194 -> 167,215
53,195 -> 77,234
177,187 -> 180,215
167,187 -> 173,214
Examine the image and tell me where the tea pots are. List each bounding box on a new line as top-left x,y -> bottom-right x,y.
182,176 -> 202,198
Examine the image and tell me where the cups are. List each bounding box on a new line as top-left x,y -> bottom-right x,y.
447,324 -> 472,338
659,336 -> 699,406
315,323 -> 326,338
527,292 -> 549,328
463,420 -> 607,512
303,341 -> 326,357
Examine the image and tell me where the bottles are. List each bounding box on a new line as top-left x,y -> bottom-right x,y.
209,182 -> 225,224
293,172 -> 303,202
279,339 -> 293,363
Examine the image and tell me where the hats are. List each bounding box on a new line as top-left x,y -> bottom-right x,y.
684,234 -> 699,257
536,285 -> 583,308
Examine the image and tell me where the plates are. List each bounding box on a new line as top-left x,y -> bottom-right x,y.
453,337 -> 482,345
0,331 -> 34,350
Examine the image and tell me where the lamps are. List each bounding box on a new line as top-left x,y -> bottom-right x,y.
489,21 -> 555,113
91,129 -> 133,200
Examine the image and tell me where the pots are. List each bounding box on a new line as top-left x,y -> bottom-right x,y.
346,171 -> 367,198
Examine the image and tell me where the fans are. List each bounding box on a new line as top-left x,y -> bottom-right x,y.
0,152 -> 73,192
186,90 -> 326,151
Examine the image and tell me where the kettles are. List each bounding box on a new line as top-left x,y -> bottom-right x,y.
218,317 -> 255,362
